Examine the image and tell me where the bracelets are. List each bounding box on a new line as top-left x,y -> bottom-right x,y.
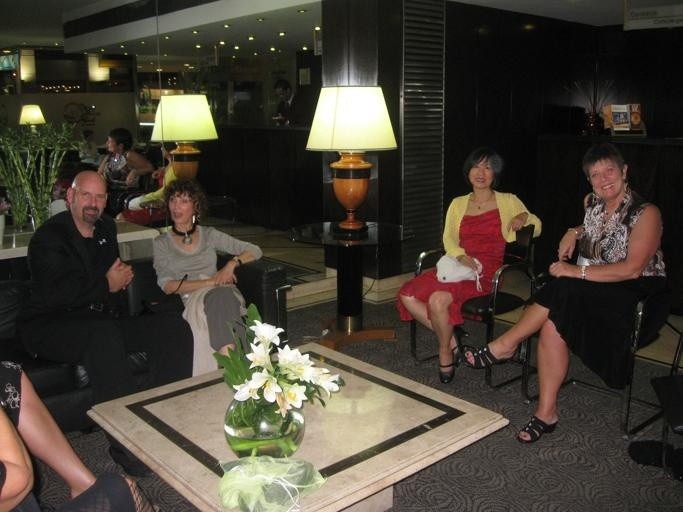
233,256 -> 242,267
581,265 -> 586,280
567,228 -> 578,235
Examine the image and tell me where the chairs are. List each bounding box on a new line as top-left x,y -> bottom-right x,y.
44,147 -> 84,187
650,317 -> 683,466
412,222 -> 540,389
521,236 -> 681,438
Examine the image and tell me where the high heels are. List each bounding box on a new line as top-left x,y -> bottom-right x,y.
438,326 -> 470,384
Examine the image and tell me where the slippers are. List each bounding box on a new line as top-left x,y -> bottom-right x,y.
465,345 -> 516,369
517,416 -> 558,443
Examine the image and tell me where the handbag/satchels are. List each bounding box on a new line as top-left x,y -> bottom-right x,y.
436,255 -> 483,283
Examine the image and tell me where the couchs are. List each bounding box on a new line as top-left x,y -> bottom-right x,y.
0,254 -> 291,434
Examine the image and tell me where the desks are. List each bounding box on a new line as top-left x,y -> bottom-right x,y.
288,216 -> 401,349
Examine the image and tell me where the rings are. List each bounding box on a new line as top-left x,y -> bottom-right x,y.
553,262 -> 555,266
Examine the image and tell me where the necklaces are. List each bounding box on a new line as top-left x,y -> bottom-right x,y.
604,201 -> 611,216
172,219 -> 198,245
472,192 -> 493,209
114,157 -> 118,162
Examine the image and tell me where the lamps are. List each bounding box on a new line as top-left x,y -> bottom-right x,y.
18,49 -> 36,83
85,49 -> 110,81
301,82 -> 398,241
149,93 -> 220,180
18,104 -> 47,136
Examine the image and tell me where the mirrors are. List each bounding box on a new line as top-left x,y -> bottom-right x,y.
1,2 -> 349,300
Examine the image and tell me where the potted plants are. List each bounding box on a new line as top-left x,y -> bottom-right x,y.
574,60 -> 620,137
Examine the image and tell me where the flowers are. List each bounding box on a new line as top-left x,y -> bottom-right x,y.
210,303 -> 347,434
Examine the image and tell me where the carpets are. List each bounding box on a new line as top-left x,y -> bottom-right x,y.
2,295 -> 683,512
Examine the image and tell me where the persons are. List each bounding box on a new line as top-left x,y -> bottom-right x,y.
394,145 -> 544,384
271,77 -> 310,128
14,171 -> 147,477
152,178 -> 263,378
461,141 -> 673,444
1,360 -> 155,512
151,142 -> 177,189
95,127 -> 154,188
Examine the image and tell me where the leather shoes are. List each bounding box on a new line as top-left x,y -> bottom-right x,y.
109,447 -> 154,478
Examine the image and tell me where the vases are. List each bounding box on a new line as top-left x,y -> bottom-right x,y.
223,395 -> 310,458
